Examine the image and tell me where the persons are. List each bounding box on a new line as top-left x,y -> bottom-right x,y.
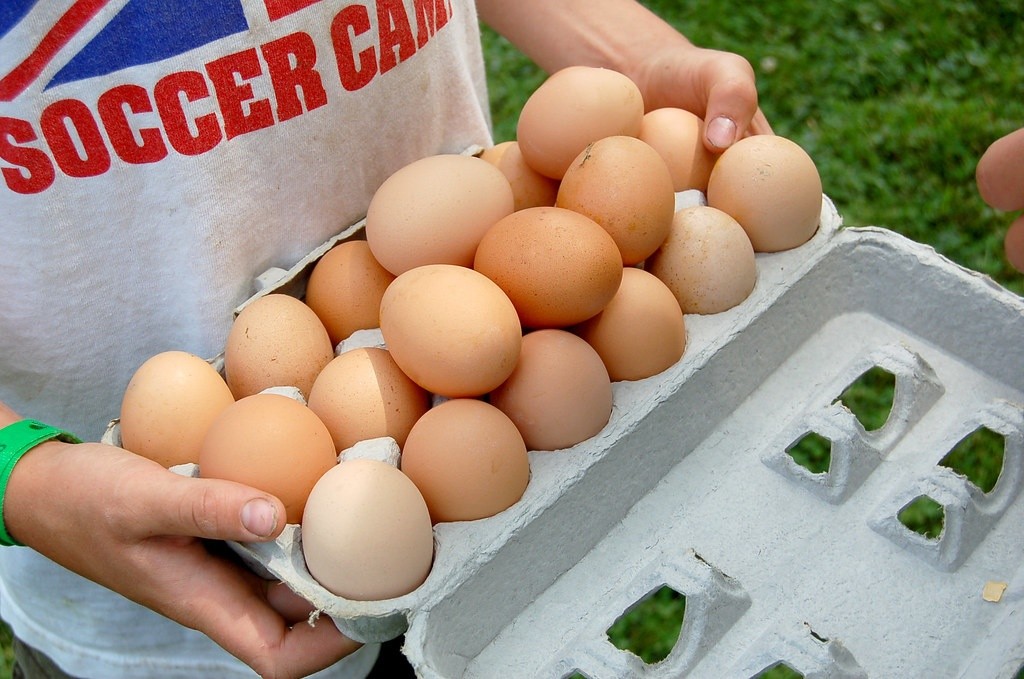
0,0 -> 776,679
976,127 -> 1024,275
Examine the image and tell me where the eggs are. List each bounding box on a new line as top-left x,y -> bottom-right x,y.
123,66 -> 824,602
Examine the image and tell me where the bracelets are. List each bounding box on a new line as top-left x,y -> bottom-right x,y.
0,419 -> 84,546
